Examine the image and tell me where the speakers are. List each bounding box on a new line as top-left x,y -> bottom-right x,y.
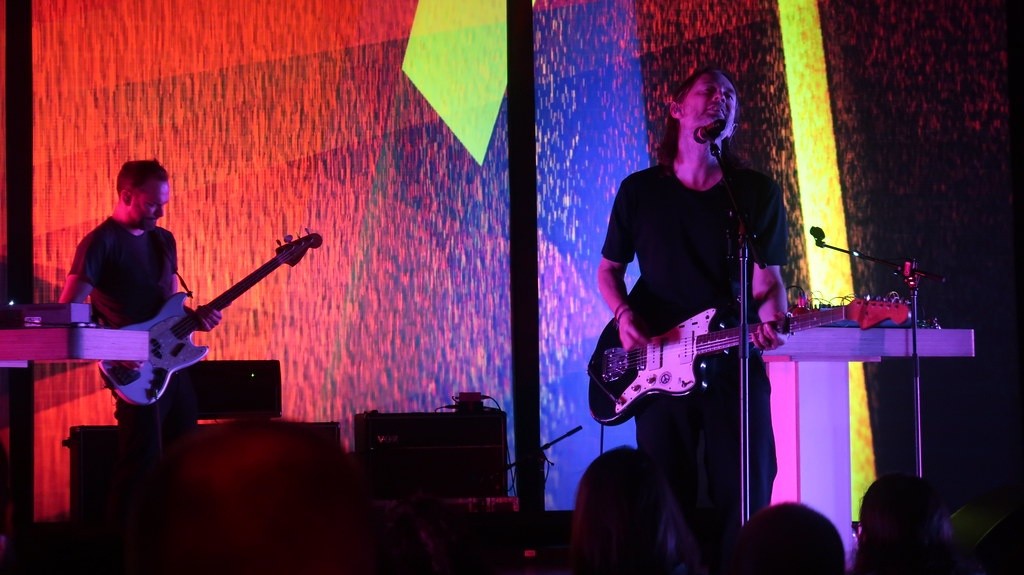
67,424 -> 122,528
193,361 -> 283,421
355,411 -> 508,500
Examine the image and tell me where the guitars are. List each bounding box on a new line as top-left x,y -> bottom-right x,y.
98,229 -> 323,406
587,293 -> 914,427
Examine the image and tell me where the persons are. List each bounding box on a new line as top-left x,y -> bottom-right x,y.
730,500 -> 846,575
570,445 -> 705,575
850,473 -> 985,575
58,161 -> 222,527
599,69 -> 787,575
122,417 -> 379,575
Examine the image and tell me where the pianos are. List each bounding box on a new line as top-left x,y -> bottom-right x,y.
754,304 -> 976,363
0,302 -> 150,363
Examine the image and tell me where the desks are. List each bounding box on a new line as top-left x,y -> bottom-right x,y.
754,329 -> 974,570
0,329 -> 149,528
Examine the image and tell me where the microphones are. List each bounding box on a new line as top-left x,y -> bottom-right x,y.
693,119 -> 727,144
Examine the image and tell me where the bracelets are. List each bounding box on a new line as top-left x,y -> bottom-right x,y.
614,303 -> 630,320
